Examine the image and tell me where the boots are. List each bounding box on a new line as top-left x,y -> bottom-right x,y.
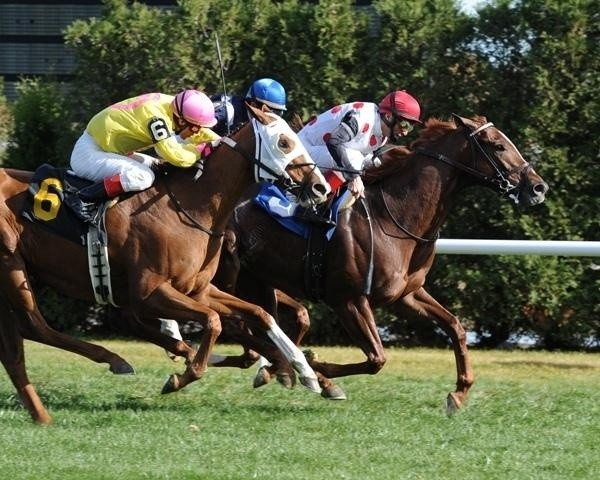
63,178 -> 109,229
293,193 -> 337,226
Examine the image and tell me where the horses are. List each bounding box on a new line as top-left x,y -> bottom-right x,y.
0,100 -> 331,425
237,113 -> 550,418
104,114 -> 347,400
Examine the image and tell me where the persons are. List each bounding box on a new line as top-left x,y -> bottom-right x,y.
296,90 -> 423,217
62,89 -> 222,220
209,77 -> 287,136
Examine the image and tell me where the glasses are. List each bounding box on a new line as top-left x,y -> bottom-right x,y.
175,91 -> 200,133
251,84 -> 284,117
390,91 -> 414,133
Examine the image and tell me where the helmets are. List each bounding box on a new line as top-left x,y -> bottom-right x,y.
172,90 -> 218,128
378,90 -> 423,132
245,78 -> 288,111
